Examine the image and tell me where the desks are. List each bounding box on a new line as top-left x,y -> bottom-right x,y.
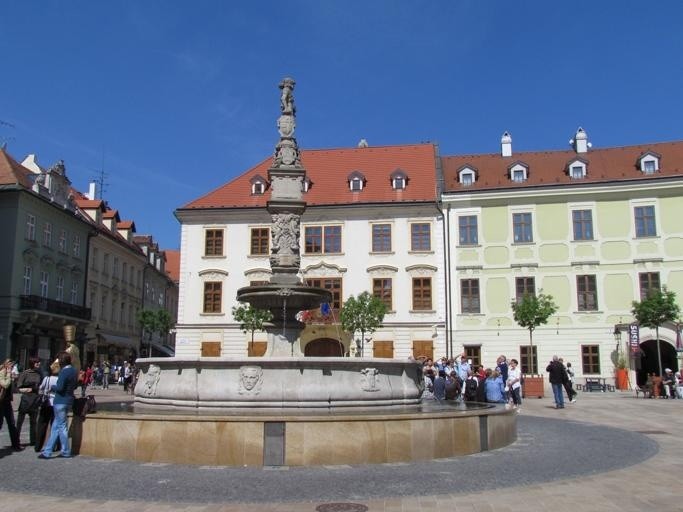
586,378 -> 606,392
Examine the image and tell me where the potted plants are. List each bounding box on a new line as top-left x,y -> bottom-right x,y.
616,355 -> 628,389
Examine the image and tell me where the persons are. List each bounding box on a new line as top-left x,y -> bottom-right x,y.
661,367 -> 679,399
0,351 -> 134,459
422,351 -> 523,415
545,355 -> 575,409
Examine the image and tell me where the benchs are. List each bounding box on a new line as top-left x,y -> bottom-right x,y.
636,389 -> 677,399
576,382 -> 615,392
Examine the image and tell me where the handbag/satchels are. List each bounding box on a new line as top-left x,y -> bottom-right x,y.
74,395 -> 96,416
36,394 -> 49,406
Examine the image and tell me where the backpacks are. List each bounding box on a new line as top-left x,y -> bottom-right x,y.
466,376 -> 476,397
